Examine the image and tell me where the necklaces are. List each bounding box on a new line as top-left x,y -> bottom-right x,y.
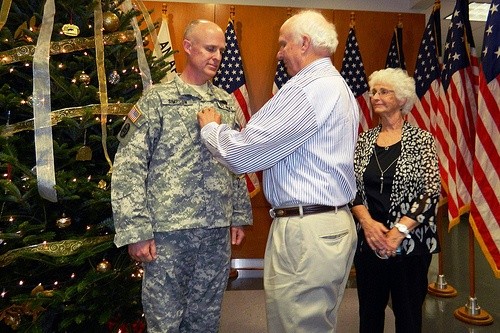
382,126 -> 394,151
374,146 -> 401,194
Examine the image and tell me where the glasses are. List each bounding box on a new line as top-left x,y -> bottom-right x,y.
369,89 -> 395,97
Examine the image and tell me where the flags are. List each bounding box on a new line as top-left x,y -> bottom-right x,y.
157,0 -> 500,277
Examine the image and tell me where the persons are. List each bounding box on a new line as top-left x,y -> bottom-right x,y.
348,67 -> 441,333
196,11 -> 359,333
111,18 -> 254,333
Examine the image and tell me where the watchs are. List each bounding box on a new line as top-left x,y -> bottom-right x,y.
395,223 -> 412,238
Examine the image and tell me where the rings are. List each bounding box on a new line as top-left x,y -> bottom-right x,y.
386,251 -> 390,254
392,252 -> 396,253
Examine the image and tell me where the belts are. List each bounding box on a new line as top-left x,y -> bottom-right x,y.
273,203 -> 346,218
